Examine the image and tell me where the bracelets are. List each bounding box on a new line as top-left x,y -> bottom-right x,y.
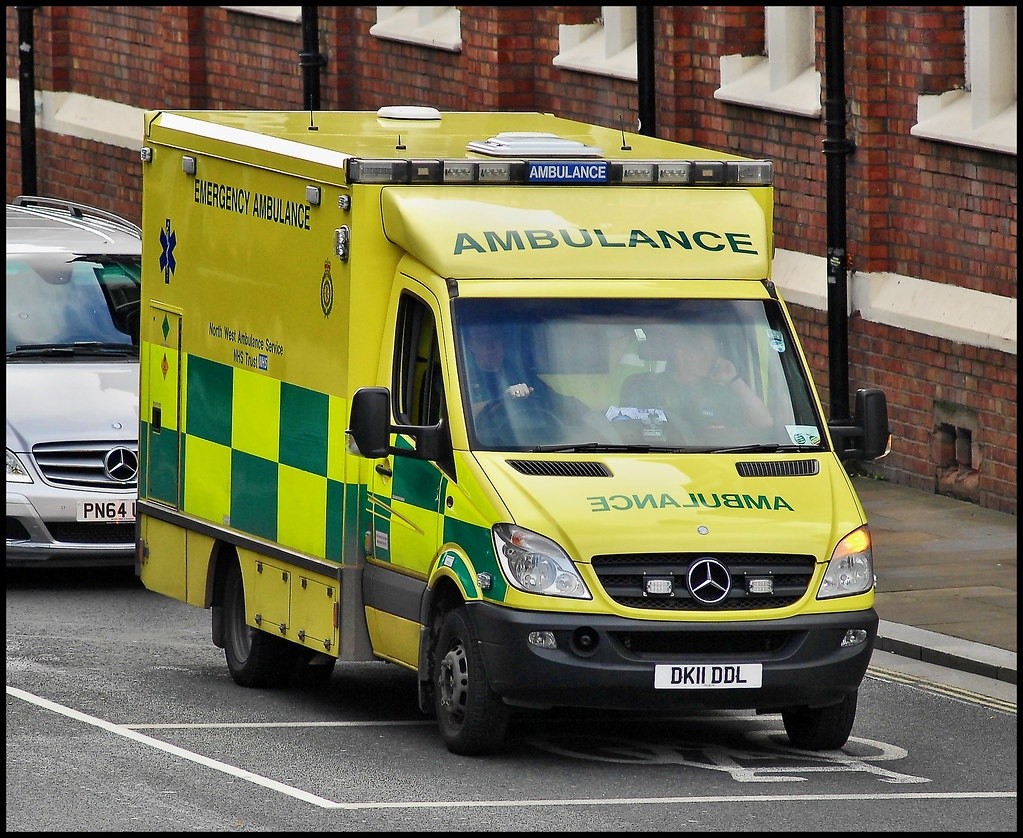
726,372 -> 743,390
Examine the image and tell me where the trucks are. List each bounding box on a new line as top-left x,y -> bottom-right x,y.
134,100 -> 885,767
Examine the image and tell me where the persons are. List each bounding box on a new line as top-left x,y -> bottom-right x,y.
466,310 -> 564,425
622,315 -> 774,450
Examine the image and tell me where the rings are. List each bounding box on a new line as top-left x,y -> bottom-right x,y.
513,391 -> 521,396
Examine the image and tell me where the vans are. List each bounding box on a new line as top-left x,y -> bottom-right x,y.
2,194 -> 146,580
